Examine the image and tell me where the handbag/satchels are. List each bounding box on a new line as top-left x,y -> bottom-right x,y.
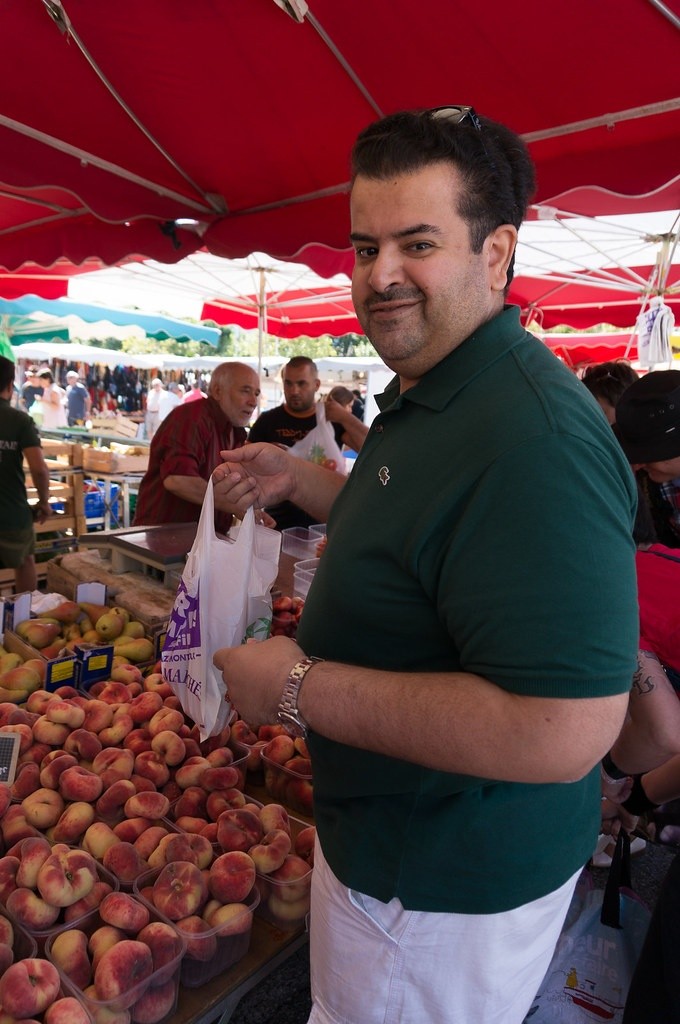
162,473 -> 282,742
288,402 -> 348,475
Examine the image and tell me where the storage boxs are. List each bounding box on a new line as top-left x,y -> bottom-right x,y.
282,527 -> 324,560
8,579 -> 169,689
214,815 -> 315,928
51,480 -> 119,529
21,845 -> 121,959
294,558 -> 320,597
229,523 -> 282,566
308,524 -> 326,554
0,596 -> 78,711
106,816 -> 185,892
83,446 -> 153,474
44,894 -> 188,1024
228,742 -> 251,787
133,852 -> 261,988
261,744 -> 315,818
293,567 -> 317,602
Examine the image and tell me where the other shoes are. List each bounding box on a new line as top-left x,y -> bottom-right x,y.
591,836 -> 646,867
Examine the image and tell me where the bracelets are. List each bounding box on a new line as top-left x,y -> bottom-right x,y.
621,773 -> 662,816
601,762 -> 632,784
602,751 -> 632,780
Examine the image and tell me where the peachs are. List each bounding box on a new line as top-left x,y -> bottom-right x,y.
0,659 -> 314,1024
269,597 -> 306,637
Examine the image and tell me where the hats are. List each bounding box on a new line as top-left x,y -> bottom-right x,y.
611,370 -> 680,464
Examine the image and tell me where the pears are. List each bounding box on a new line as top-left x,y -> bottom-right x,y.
0,601 -> 154,705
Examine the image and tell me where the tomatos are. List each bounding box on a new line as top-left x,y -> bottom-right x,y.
325,459 -> 337,471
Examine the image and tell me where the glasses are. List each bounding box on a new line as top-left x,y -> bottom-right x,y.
426,105 -> 492,165
585,366 -> 627,390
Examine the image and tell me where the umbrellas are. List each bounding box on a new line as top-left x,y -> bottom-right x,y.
0,0 -> 680,419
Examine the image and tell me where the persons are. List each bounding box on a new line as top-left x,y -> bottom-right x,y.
10,365 -> 208,440
351,389 -> 365,423
0,355 -> 52,594
212,105 -> 639,1024
581,361 -> 680,1024
131,356 -> 369,536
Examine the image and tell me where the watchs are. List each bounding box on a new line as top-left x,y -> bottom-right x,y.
277,656 -> 325,741
